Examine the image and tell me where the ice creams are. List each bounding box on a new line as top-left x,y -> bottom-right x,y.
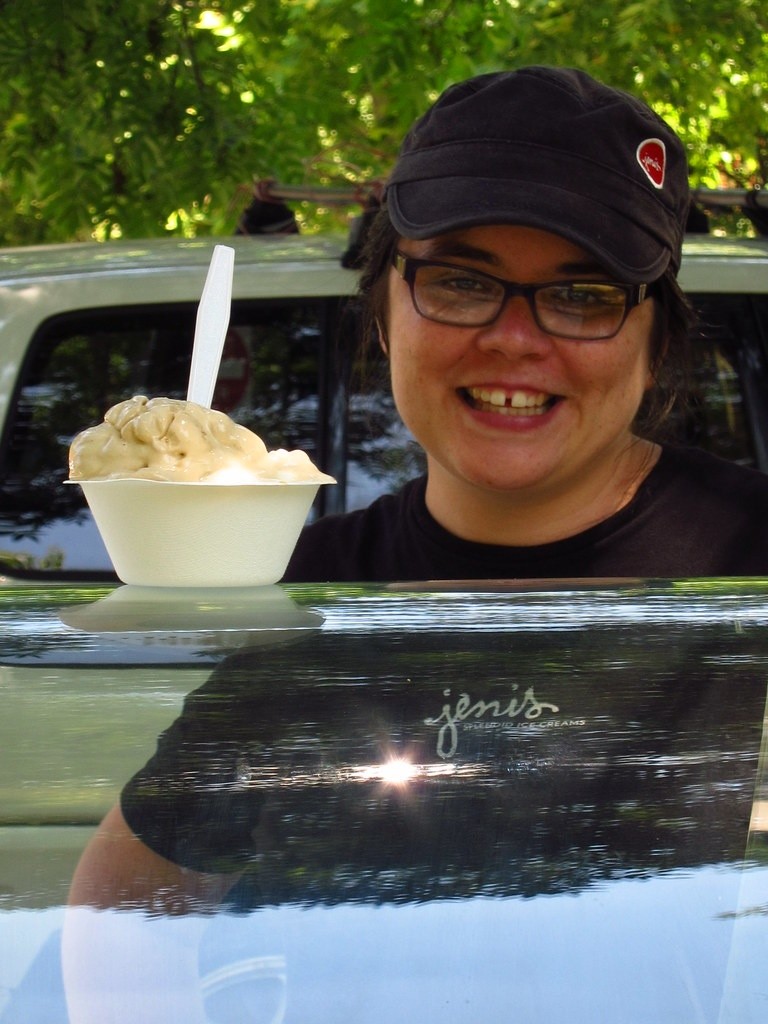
72,392 -> 338,485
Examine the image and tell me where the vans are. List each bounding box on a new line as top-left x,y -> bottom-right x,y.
2,237 -> 764,887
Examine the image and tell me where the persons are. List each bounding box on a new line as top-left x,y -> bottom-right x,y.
60,66 -> 767,1023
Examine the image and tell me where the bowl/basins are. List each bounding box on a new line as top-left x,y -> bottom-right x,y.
62,473 -> 338,589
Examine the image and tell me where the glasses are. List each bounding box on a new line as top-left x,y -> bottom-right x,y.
384,247 -> 678,343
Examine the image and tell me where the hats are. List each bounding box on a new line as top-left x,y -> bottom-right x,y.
388,65 -> 690,285
